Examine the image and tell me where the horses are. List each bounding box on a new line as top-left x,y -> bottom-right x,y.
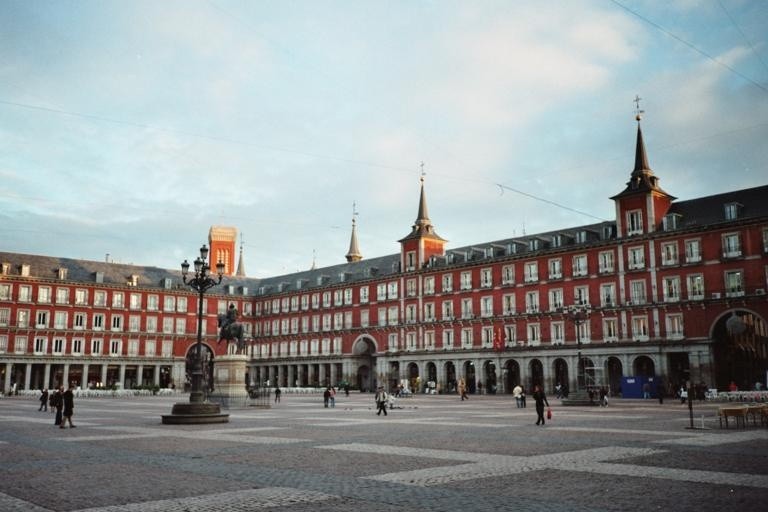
215,312 -> 247,354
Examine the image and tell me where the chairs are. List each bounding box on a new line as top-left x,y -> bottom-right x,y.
704,391 -> 768,402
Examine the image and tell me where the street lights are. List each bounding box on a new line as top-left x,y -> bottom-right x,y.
180,245 -> 224,404
561,304 -> 591,389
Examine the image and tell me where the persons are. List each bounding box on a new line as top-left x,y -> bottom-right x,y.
274,385 -> 282,403
519,385 -> 527,408
640,379 -> 713,404
596,385 -> 610,409
512,383 -> 523,408
327,386 -> 337,407
532,384 -> 549,426
222,303 -> 239,329
53,386 -> 60,397
555,381 -> 564,399
343,383 -> 350,396
324,388 -> 331,408
398,377 -> 483,401
60,383 -> 77,428
737,377 -> 767,391
728,379 -> 737,392
54,385 -> 65,425
589,389 -> 596,406
49,390 -> 56,412
374,385 -> 389,416
38,385 -> 48,412
